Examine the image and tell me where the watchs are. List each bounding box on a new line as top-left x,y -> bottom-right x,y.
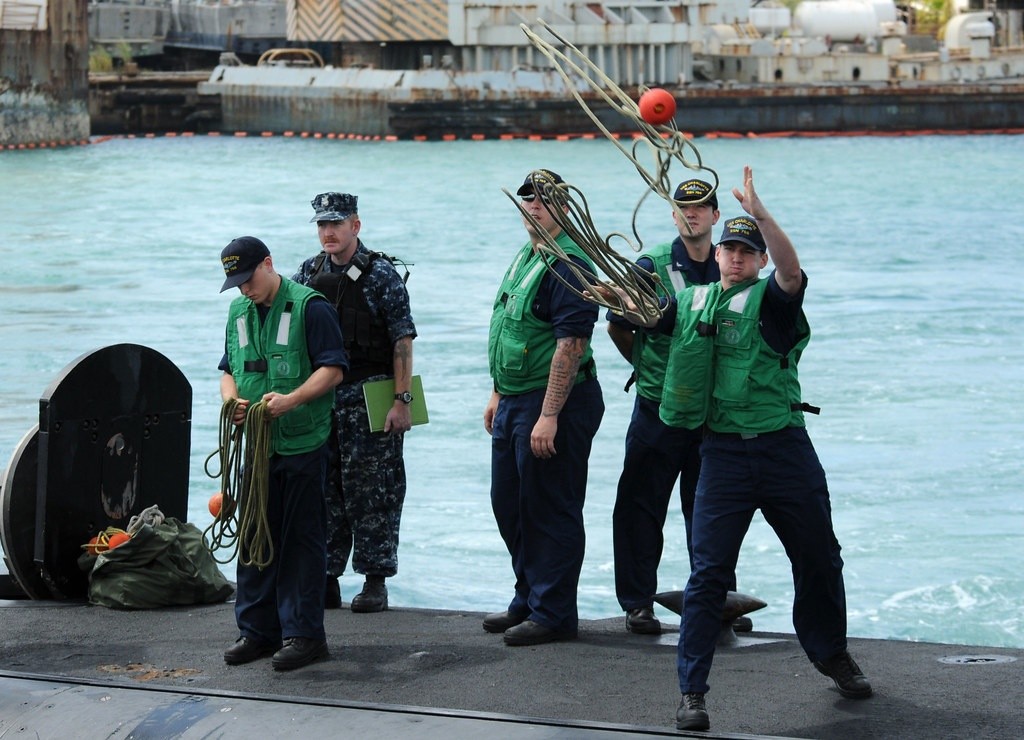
395,391 -> 413,404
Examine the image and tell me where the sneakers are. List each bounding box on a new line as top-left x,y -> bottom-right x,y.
484,608 -> 527,633
625,604 -> 662,633
324,593 -> 342,610
732,614 -> 753,632
504,617 -> 578,645
676,691 -> 711,731
351,581 -> 388,612
272,633 -> 328,670
223,635 -> 272,663
813,649 -> 872,699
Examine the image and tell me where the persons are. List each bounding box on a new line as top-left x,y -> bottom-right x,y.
583,165 -> 873,731
483,168 -> 604,646
288,192 -> 418,612
219,236 -> 351,668
606,178 -> 754,635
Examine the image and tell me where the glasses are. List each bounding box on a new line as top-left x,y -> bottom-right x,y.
520,187 -> 552,204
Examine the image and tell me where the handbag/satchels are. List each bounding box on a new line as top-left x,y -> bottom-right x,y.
78,517 -> 235,609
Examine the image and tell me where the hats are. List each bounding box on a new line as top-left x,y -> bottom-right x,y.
311,193 -> 358,221
716,215 -> 766,253
517,169 -> 568,203
220,236 -> 270,293
673,179 -> 718,209
